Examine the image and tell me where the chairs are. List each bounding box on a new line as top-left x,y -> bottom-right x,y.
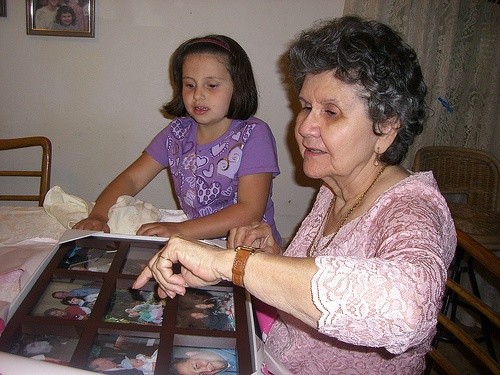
412,146 -> 500,348
0,136 -> 52,207
427,227 -> 500,375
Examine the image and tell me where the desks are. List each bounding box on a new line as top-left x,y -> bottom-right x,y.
0,210 -> 259,375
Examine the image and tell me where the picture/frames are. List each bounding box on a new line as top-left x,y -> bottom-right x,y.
26,0 -> 95,38
0,229 -> 255,375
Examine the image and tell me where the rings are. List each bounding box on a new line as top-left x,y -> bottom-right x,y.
158,256 -> 175,266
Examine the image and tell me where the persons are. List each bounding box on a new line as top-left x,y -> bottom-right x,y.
184,295 -> 235,331
17,338 -> 80,363
45,288 -> 101,321
35,0 -> 89,32
89,349 -> 158,375
71,35 -> 283,247
169,349 -> 238,375
123,304 -> 163,323
130,14 -> 457,375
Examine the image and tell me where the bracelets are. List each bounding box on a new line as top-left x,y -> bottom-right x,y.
232,245 -> 265,288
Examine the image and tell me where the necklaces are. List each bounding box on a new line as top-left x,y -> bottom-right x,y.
306,164 -> 389,258
